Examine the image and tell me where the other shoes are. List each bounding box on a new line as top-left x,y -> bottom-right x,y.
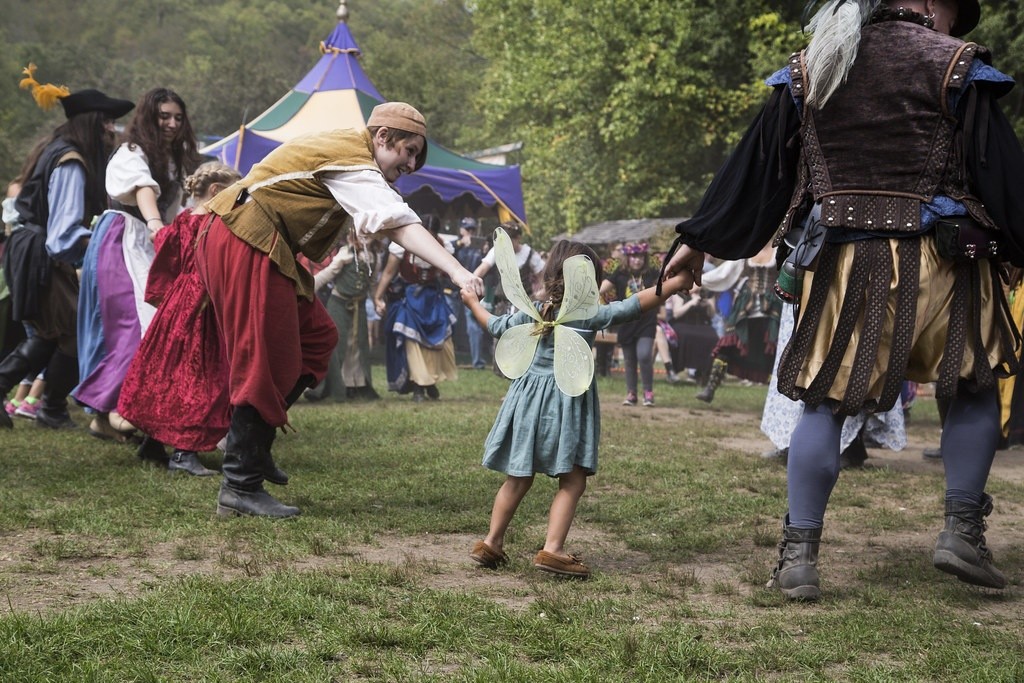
428,385 -> 440,399
108,411 -> 134,431
469,540 -> 510,567
536,549 -> 591,576
414,387 -> 426,400
89,420 -> 126,441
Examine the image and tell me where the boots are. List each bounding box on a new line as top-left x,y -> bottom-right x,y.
695,360 -> 727,402
932,489 -> 1008,588
0,338 -> 41,428
34,353 -> 75,430
258,428 -> 288,484
216,404 -> 301,518
767,512 -> 822,599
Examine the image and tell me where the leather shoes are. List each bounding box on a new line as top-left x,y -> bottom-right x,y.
170,447 -> 221,478
924,447 -> 945,460
137,434 -> 171,470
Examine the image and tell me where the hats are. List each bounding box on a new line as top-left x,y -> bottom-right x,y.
367,102 -> 428,138
55,88 -> 135,119
460,217 -> 479,229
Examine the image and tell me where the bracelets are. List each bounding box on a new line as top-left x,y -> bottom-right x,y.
147,217 -> 162,222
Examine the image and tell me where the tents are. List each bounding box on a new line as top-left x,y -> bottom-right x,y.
195,0 -> 525,230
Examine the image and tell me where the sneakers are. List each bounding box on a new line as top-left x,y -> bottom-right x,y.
6,399 -> 22,415
644,392 -> 654,405
15,397 -> 42,418
623,393 -> 637,406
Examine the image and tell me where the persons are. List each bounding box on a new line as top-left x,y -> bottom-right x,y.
596,238 -> 780,407
0,88 -> 550,519
661,0 -> 1024,605
460,241 -> 695,579
